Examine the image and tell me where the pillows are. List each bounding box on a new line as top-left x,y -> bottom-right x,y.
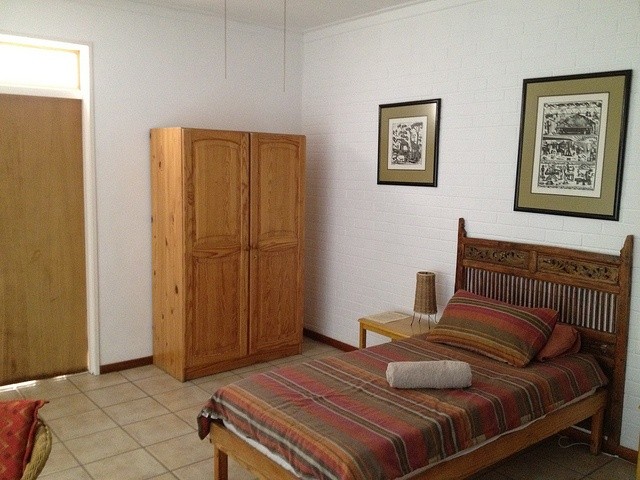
1,397 -> 49,480
537,322 -> 582,363
426,289 -> 560,369
386,359 -> 473,391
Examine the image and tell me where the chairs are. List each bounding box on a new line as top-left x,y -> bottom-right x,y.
20,415 -> 53,480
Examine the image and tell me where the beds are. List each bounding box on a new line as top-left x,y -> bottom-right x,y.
194,214 -> 635,480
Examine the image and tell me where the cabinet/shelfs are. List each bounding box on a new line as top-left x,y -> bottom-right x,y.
148,122 -> 307,385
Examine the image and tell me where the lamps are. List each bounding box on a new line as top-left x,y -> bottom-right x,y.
409,270 -> 438,331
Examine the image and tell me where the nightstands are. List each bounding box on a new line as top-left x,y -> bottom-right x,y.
358,310 -> 437,350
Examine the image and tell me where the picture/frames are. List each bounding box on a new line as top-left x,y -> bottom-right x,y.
376,98 -> 444,188
513,68 -> 634,223
